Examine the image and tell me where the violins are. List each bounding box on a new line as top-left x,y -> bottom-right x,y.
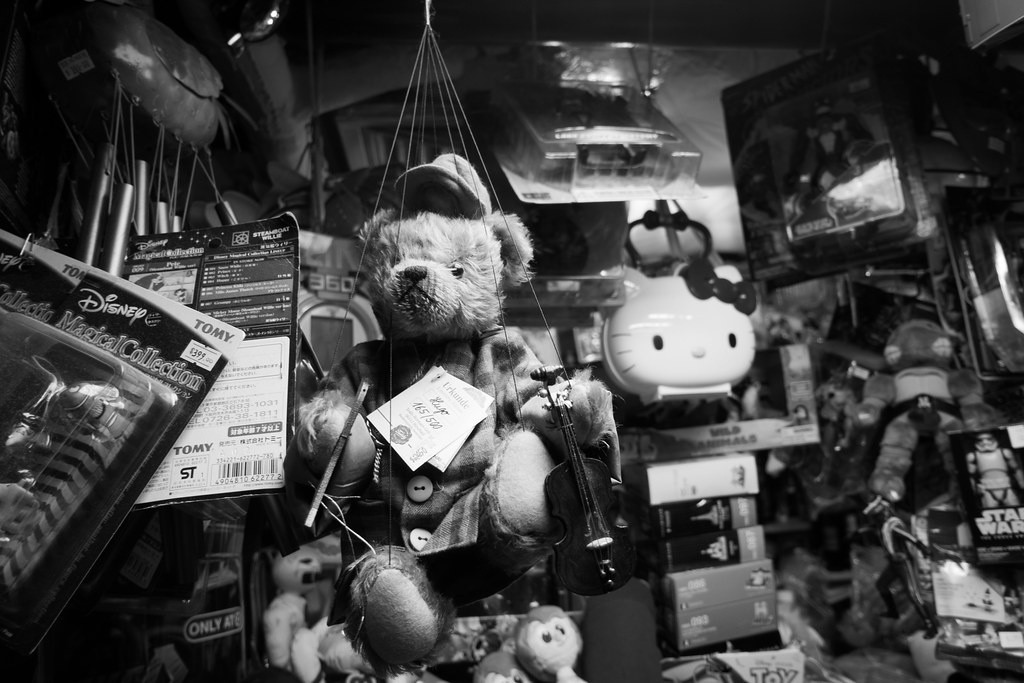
526,364 -> 643,597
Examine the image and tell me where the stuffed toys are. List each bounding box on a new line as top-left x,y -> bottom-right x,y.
805,372 -> 866,488
851,317 -> 984,505
263,204 -> 595,683
